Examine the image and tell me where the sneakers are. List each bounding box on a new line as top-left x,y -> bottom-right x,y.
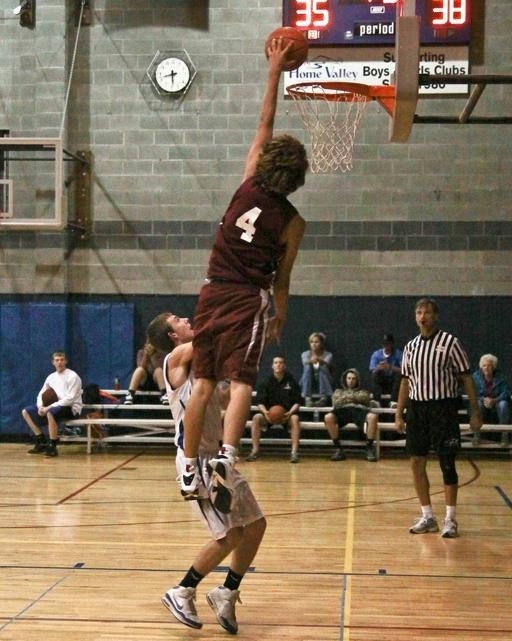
27,445 -> 60,458
206,584 -> 243,633
179,467 -> 208,500
441,518 -> 461,538
205,457 -> 235,513
368,399 -> 398,409
409,516 -> 439,533
246,448 -> 377,464
162,585 -> 202,629
302,397 -> 329,408
159,394 -> 169,406
122,392 -> 136,406
471,430 -> 511,449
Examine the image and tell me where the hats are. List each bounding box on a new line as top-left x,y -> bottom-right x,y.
382,333 -> 394,345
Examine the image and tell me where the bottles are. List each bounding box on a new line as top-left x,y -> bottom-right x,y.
114,376 -> 120,391
313,410 -> 319,422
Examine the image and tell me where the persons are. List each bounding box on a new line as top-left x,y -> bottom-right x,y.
395,298 -> 483,538
124,344 -> 169,405
298,332 -> 333,407
246,354 -> 302,463
324,367 -> 378,462
467,353 -> 511,447
22,351 -> 84,457
146,312 -> 267,634
179,35 -> 308,512
368,333 -> 403,408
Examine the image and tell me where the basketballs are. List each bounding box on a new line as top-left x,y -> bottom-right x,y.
269,405 -> 285,423
43,387 -> 58,405
265,26 -> 307,71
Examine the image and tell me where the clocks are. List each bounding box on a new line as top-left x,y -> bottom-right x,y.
146,49 -> 195,97
